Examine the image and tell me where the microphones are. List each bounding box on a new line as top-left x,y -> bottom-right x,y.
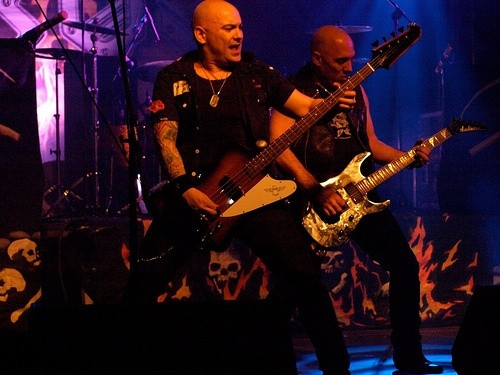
145,6 -> 160,41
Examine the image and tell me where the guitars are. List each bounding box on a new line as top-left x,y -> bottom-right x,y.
301,116 -> 489,251
161,22 -> 424,259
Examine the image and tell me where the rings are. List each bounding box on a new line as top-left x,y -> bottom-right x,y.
423,161 -> 429,166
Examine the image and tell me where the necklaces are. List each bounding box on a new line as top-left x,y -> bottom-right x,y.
198,58 -> 228,108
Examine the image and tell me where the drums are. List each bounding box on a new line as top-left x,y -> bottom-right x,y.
105,123 -> 162,169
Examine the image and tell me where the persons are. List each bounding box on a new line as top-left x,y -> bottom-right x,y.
264,24 -> 444,375
430,142 -> 494,303
148,0 -> 357,375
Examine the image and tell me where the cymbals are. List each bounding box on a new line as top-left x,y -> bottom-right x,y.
35,48 -> 93,58
135,60 -> 175,83
62,21 -> 130,36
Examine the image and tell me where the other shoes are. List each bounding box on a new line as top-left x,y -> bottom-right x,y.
394,353 -> 444,374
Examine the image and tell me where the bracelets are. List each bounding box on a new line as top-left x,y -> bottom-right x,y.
173,174 -> 194,195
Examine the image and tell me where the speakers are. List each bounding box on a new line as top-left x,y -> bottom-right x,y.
453,284 -> 500,375
0,291 -> 301,374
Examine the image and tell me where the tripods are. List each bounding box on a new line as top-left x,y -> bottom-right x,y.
41,32 -> 131,220
